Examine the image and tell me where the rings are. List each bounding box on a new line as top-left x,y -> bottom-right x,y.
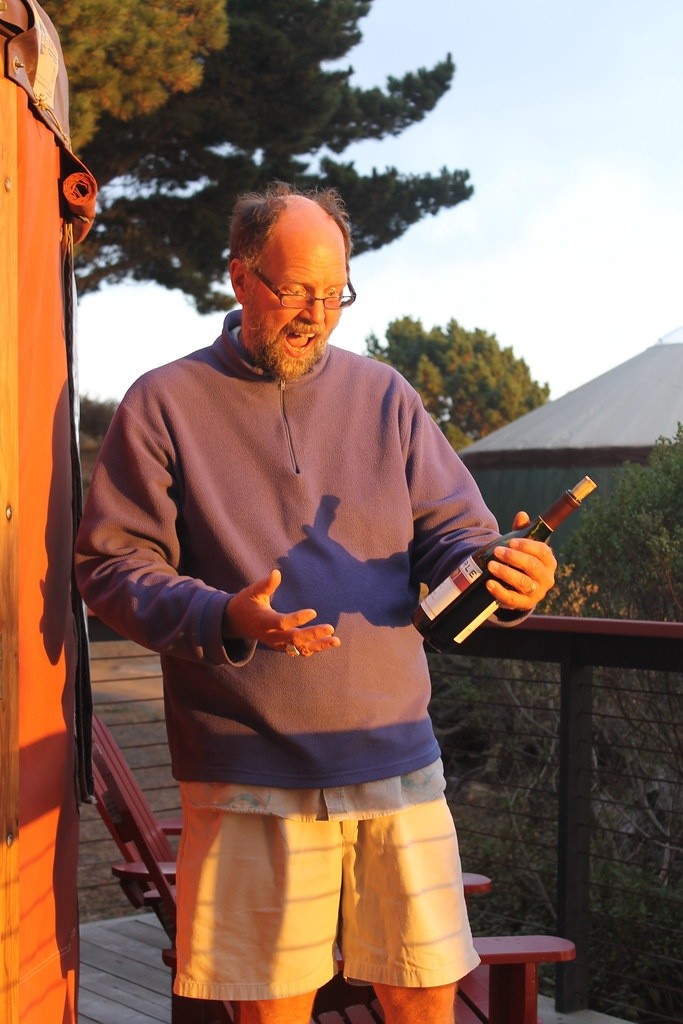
525,582 -> 535,593
285,643 -> 300,658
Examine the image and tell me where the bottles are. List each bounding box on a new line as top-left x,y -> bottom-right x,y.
408,475 -> 599,656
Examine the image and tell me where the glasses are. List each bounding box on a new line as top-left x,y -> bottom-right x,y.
251,268 -> 356,310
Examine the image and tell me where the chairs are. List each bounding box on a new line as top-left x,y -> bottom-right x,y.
73,701 -> 581,1023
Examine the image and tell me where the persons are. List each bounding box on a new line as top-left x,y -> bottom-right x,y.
71,182 -> 559,1023
